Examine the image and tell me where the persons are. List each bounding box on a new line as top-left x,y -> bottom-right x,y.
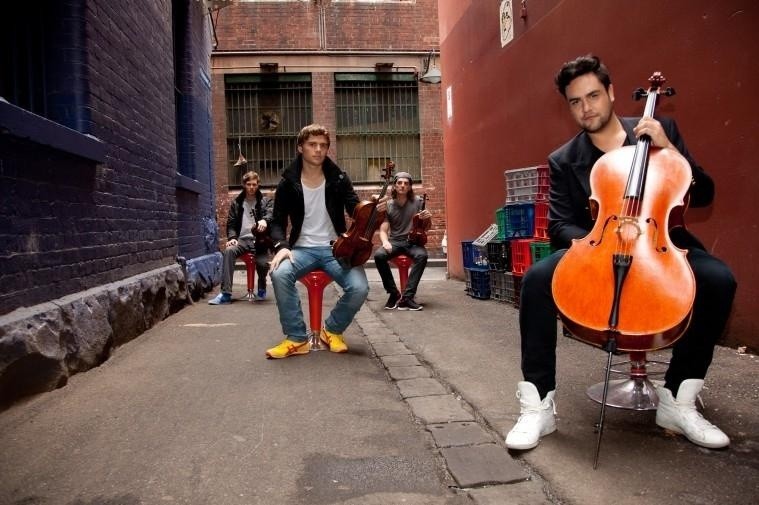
264,122 -> 389,359
373,171 -> 432,312
204,169 -> 276,304
502,51 -> 730,450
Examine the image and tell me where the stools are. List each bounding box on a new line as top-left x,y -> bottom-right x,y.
586,347 -> 670,412
390,257 -> 414,304
296,271 -> 335,353
238,254 -> 262,302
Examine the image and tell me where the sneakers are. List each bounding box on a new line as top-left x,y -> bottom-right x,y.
398,298 -> 423,311
207,292 -> 232,305
320,325 -> 348,353
266,340 -> 310,359
256,288 -> 266,300
383,293 -> 402,310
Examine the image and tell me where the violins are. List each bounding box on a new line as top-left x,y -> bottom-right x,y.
249,209 -> 271,256
332,161 -> 395,269
407,193 -> 429,247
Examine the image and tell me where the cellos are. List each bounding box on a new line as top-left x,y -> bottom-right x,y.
551,72 -> 696,353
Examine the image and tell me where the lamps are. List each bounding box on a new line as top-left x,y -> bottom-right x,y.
419,48 -> 443,85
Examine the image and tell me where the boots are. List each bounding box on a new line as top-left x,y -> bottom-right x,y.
504,381 -> 558,451
654,378 -> 730,448
